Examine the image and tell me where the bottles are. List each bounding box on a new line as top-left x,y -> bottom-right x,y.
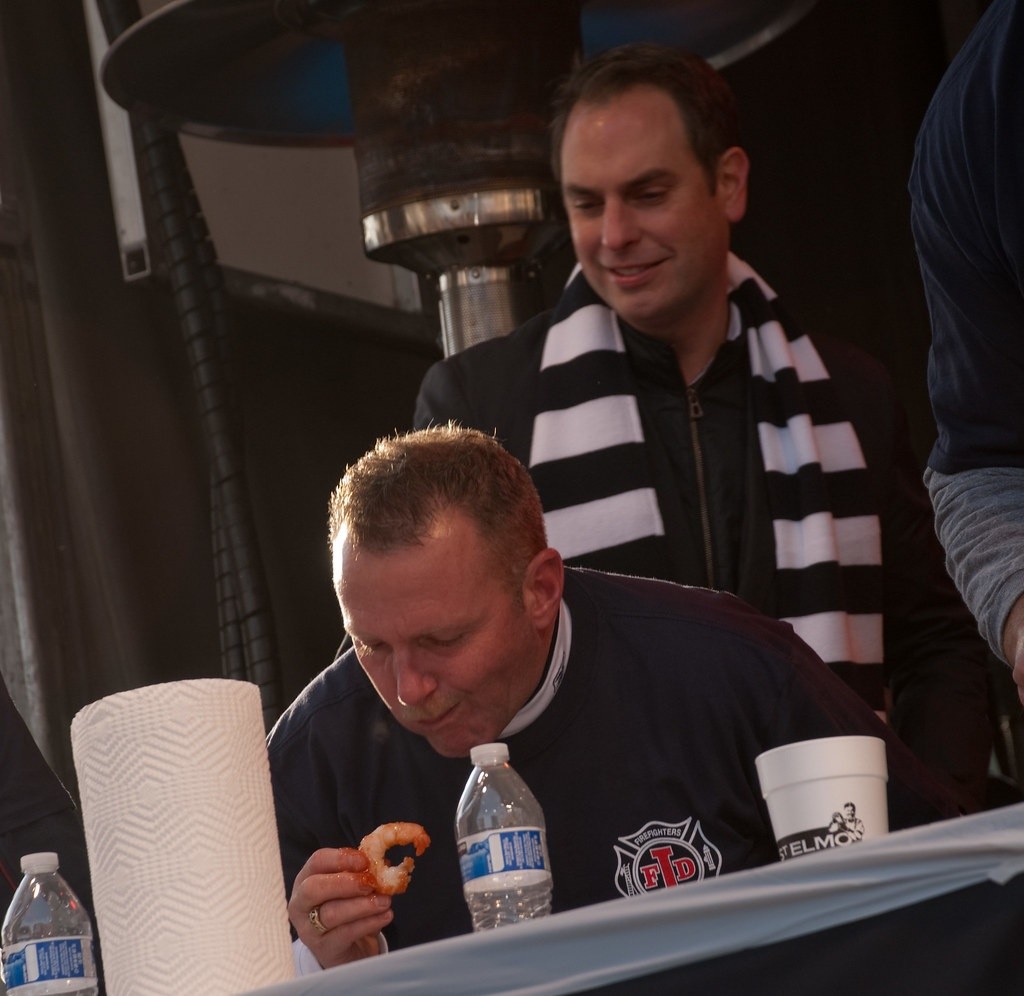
455,743 -> 554,933
2,852 -> 100,996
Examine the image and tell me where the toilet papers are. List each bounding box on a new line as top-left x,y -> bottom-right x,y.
69,678 -> 298,996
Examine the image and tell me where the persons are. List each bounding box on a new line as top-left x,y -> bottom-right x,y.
909,0 -> 1024,708
411,40 -> 991,826
267,421 -> 958,977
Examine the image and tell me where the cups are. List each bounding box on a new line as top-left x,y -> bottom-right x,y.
755,735 -> 888,863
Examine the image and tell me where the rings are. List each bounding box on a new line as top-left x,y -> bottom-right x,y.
308,907 -> 327,933
